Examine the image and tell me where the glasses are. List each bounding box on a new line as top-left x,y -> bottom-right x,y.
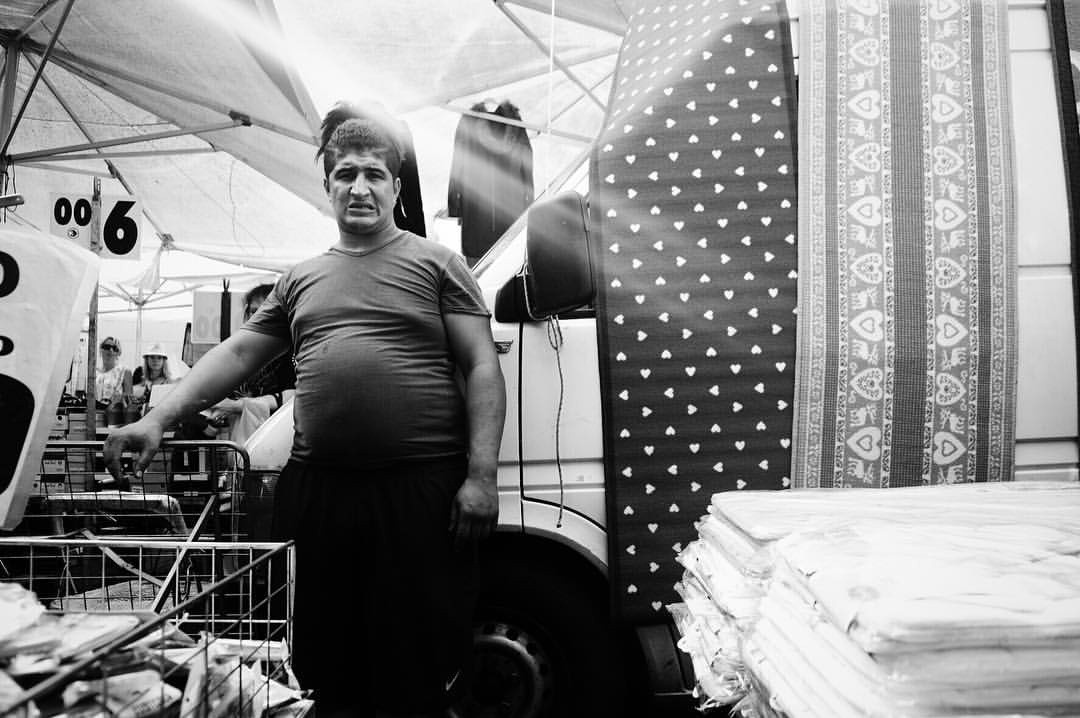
102,344 -> 119,352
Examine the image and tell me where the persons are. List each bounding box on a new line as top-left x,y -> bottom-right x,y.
101,120 -> 506,718
205,283 -> 296,422
130,345 -> 169,417
94,336 -> 132,410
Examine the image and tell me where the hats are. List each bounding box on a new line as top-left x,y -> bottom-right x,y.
143,345 -> 168,360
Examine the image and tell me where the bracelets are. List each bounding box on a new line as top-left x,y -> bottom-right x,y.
273,391 -> 283,408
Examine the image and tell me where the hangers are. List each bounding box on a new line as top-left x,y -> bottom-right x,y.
457,88 -> 522,130
